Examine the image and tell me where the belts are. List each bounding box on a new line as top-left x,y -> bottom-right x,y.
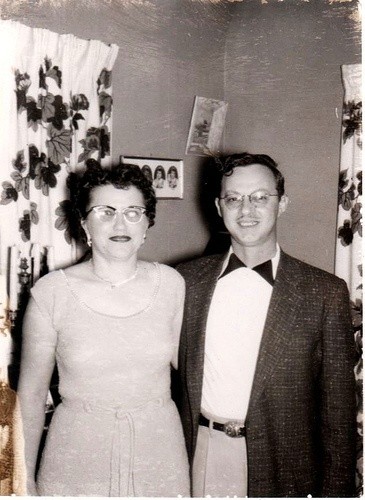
199,416 -> 245,437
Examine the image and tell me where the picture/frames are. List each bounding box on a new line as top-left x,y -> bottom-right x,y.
119,154 -> 183,199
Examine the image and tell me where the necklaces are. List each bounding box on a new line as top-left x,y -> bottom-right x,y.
88,259 -> 139,287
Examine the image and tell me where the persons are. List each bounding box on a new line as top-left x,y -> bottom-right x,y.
11,162 -> 191,498
163,153 -> 364,498
142,164 -> 179,191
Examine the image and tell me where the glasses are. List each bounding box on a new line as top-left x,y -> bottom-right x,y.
84,205 -> 146,224
219,192 -> 278,210
